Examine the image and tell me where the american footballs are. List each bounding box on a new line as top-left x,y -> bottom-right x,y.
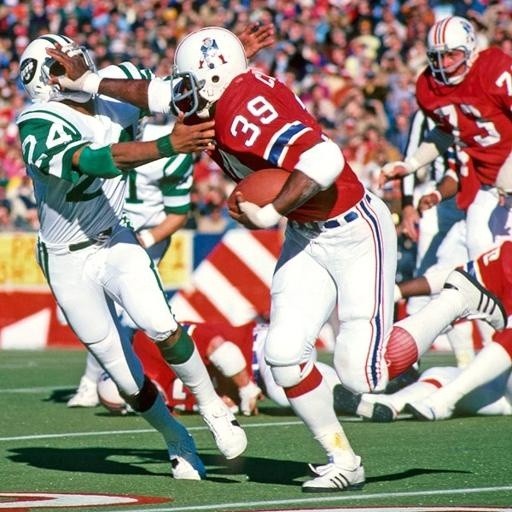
227,169 -> 291,214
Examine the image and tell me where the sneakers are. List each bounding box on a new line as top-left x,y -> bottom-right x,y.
203,408 -> 248,460
301,456 -> 365,492
444,267 -> 509,333
334,383 -> 398,423
67,373 -> 102,408
166,435 -> 207,482
408,396 -> 456,420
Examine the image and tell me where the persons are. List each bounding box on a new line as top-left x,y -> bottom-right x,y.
0,0 -> 511,419
46,27 -> 508,491
17,22 -> 274,481
67,157 -> 192,410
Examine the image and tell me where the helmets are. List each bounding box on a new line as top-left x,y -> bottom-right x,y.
425,17 -> 480,85
18,34 -> 98,105
168,26 -> 248,118
